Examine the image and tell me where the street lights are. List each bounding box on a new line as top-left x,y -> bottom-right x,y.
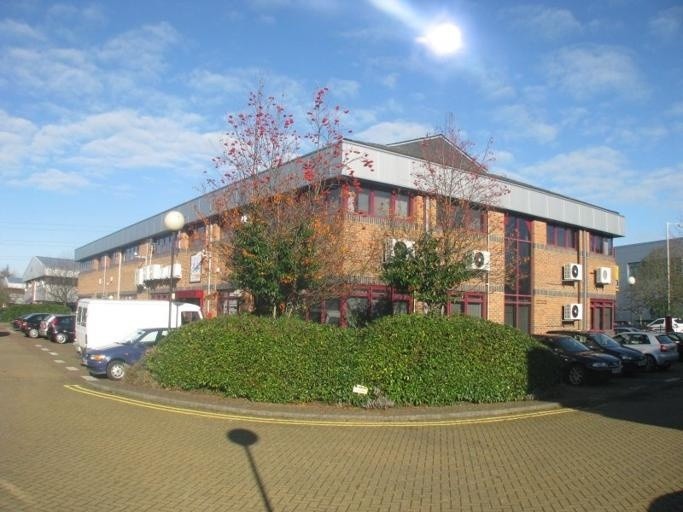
160,208 -> 185,338
661,219 -> 682,315
626,274 -> 637,323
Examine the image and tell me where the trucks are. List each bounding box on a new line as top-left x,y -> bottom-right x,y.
72,296 -> 205,355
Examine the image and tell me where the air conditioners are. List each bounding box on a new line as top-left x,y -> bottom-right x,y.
596,266 -> 612,286
466,249 -> 490,272
563,263 -> 582,321
385,239 -> 416,266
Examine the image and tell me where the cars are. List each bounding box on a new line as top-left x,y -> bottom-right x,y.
10,312 -> 74,346
85,326 -> 181,382
529,316 -> 682,388
